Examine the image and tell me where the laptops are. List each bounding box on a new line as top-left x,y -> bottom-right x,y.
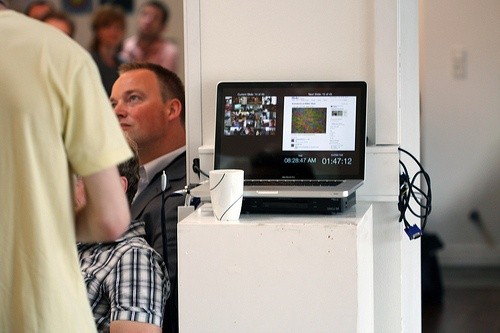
189,81 -> 367,198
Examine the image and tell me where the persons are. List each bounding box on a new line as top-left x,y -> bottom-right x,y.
0,0 -> 136,333
71,147 -> 172,333
223,95 -> 276,135
22,0 -> 186,333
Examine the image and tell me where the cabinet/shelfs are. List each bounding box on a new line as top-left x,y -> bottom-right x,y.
177,203 -> 375,333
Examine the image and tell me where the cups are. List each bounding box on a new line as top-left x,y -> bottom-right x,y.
208,169 -> 244,221
